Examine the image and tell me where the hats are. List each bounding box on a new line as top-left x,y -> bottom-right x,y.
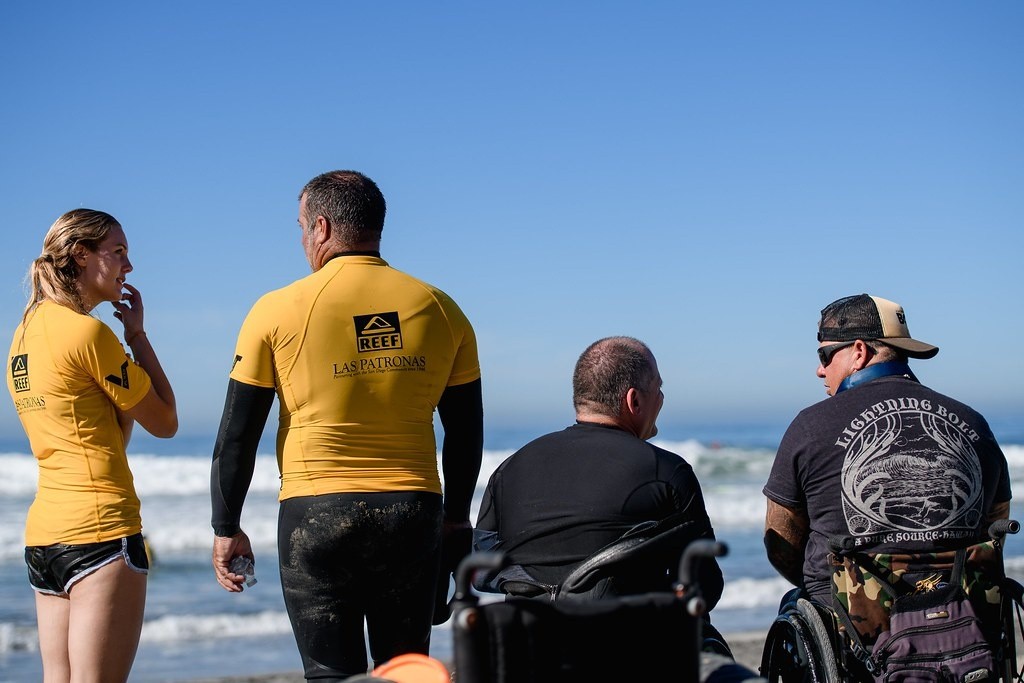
817,293 -> 939,359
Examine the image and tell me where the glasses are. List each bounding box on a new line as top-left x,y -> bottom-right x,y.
817,340 -> 877,368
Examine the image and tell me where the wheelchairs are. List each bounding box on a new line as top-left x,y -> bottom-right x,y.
448,538 -> 728,683
759,515 -> 1022,683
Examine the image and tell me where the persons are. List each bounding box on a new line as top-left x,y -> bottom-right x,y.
209,170 -> 484,683
6,208 -> 178,683
762,293 -> 1013,655
472,336 -> 724,625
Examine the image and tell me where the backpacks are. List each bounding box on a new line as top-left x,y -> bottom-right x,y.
832,547 -> 1008,683
